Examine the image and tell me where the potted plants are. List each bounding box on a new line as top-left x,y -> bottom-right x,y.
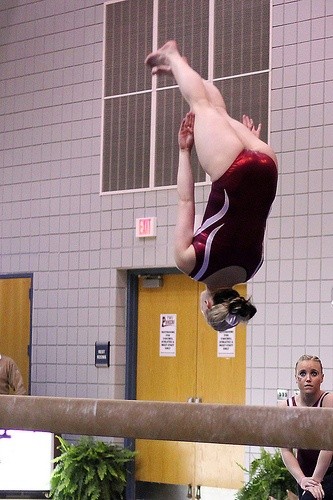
235,447 -> 299,500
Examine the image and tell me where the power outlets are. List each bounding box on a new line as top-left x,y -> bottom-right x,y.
277,390 -> 288,400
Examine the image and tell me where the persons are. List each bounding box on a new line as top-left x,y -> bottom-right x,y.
144,40 -> 278,332
0,354 -> 25,395
279,355 -> 333,500
